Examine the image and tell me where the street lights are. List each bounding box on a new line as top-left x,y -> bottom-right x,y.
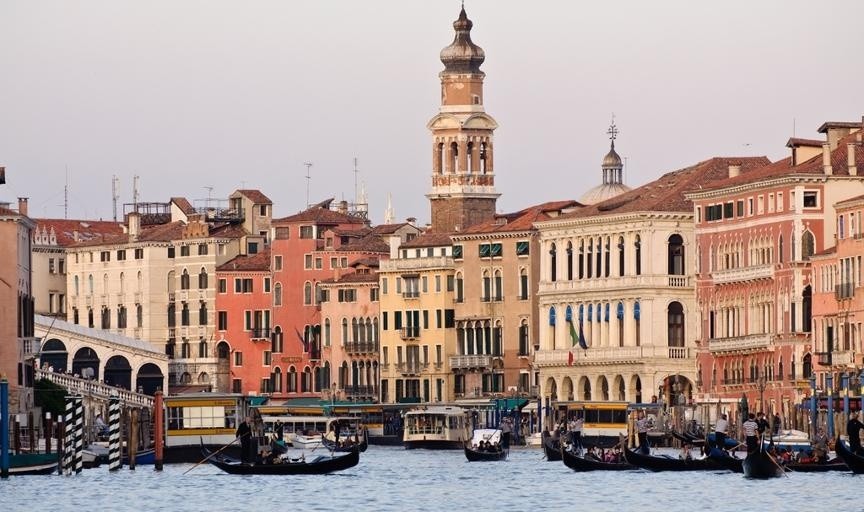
85,367 -> 94,442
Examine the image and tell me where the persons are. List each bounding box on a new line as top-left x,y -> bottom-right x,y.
636,411 -> 649,454
94,414 -> 106,437
552,415 -> 624,463
472,415 -> 530,456
256,448 -> 273,466
846,410 -> 864,454
274,420 -> 284,441
714,414 -> 729,450
236,416 -> 253,464
679,444 -> 692,460
331,420 -> 341,447
34,361 -> 128,390
742,412 -> 829,466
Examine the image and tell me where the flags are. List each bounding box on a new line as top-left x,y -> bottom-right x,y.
569,322 -> 579,347
579,323 -> 587,349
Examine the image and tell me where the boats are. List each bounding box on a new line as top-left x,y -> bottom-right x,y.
402,405 -> 476,449
463,443 -> 509,461
199,432 -> 359,475
320,425 -> 369,452
